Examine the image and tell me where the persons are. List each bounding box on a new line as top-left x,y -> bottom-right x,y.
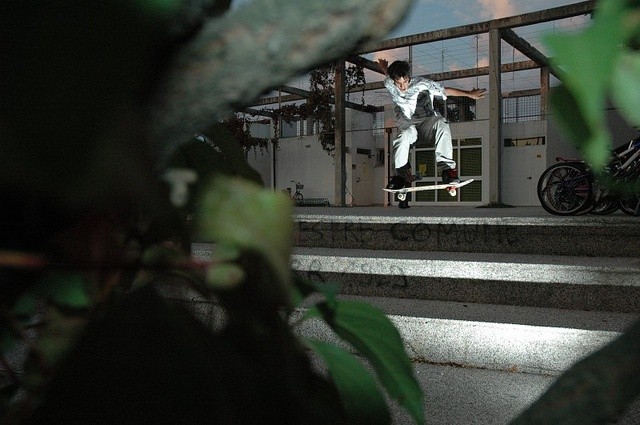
377,58 -> 488,190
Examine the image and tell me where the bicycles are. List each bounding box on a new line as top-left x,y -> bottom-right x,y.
537,139 -> 639,217
281,181 -> 304,206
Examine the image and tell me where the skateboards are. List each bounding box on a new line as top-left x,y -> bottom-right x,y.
382,178 -> 474,201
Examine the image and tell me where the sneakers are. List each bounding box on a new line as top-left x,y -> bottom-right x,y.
442,168 -> 460,183
386,176 -> 414,189
